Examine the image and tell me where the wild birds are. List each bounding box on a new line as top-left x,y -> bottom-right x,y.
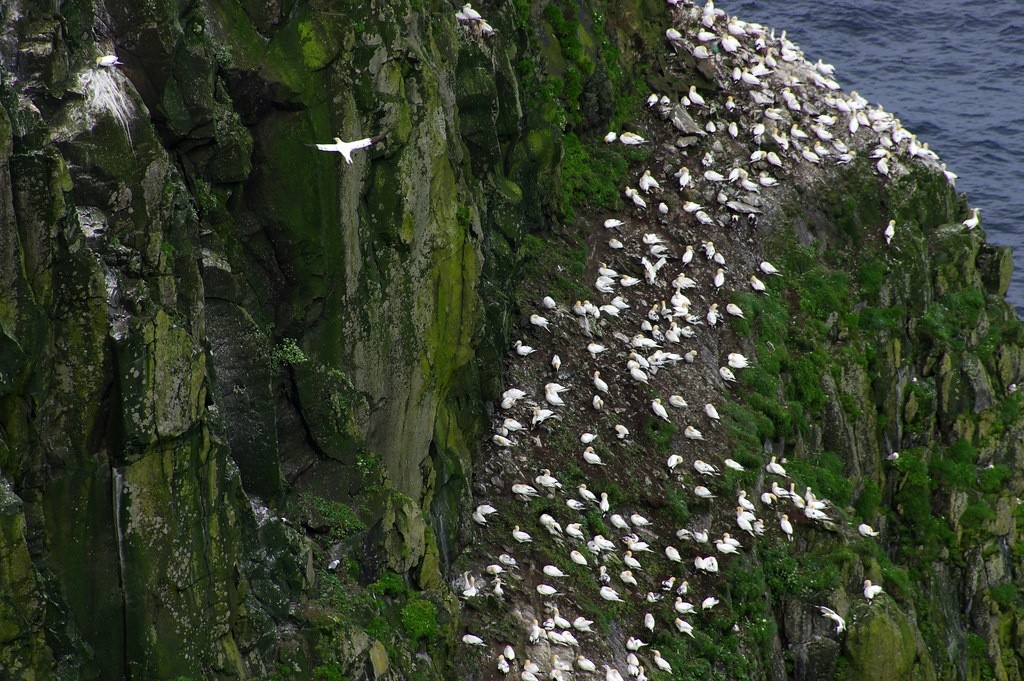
96,56 -> 125,68
305,135 -> 386,164
963,207 -> 983,229
885,220 -> 896,244
863,579 -> 885,604
328,559 -> 340,569
859,523 -> 880,538
886,451 -> 900,461
455,2 -> 493,33
461,0 -> 964,680
805,602 -> 846,635
